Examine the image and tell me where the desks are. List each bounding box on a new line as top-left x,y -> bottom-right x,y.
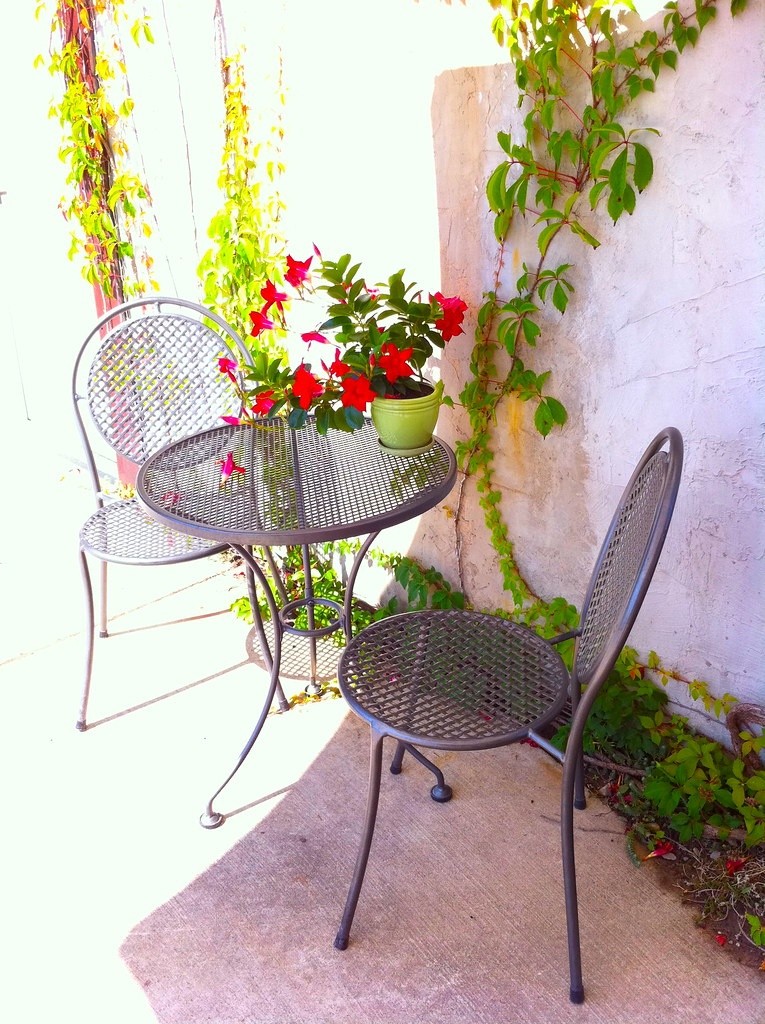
135,412 -> 459,828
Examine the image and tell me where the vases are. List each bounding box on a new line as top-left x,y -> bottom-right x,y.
368,379 -> 445,458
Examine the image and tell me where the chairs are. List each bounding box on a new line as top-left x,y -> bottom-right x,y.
335,427 -> 684,1005
75,297 -> 290,733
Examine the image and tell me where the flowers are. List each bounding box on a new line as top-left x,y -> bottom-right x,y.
192,242 -> 468,435
